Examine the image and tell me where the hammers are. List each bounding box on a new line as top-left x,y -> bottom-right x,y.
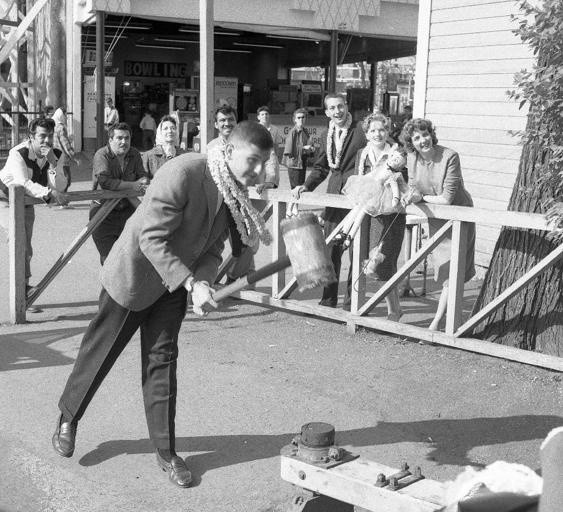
211,212 -> 338,304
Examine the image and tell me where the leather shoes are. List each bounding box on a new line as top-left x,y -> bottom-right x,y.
25,286 -> 37,299
156,448 -> 192,488
53,413 -> 77,458
26,304 -> 41,313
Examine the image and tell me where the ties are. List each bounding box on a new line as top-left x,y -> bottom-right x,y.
338,130 -> 342,140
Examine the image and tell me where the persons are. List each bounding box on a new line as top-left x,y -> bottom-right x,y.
353,113 -> 406,325
292,93 -> 367,323
45,105 -> 54,120
96,98 -> 119,142
140,110 -> 156,151
257,106 -> 283,147
50,107 -> 82,208
398,118 -> 475,345
0,116 -> 67,302
51,120 -> 272,488
144,116 -> 186,176
88,123 -> 149,265
214,105 -> 255,299
280,108 -> 316,218
